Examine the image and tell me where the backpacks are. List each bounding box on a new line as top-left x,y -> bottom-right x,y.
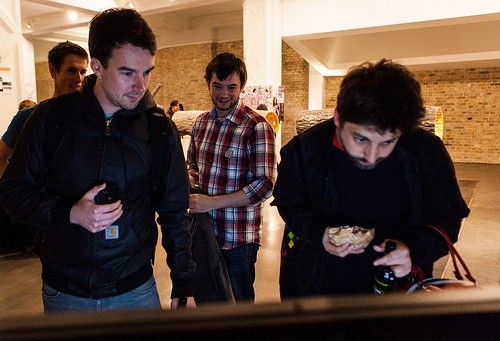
400,223 -> 482,297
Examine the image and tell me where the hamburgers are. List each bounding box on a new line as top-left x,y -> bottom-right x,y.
328,225 -> 375,250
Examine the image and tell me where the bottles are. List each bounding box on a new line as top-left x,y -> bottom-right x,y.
177,297 -> 187,309
371,241 -> 398,295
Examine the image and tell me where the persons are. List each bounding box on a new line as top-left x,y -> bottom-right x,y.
164,99 -> 181,121
0,41 -> 88,177
1,8 -> 197,314
256,104 -> 268,111
186,52 -> 278,303
271,97 -> 279,116
270,58 -> 471,301
